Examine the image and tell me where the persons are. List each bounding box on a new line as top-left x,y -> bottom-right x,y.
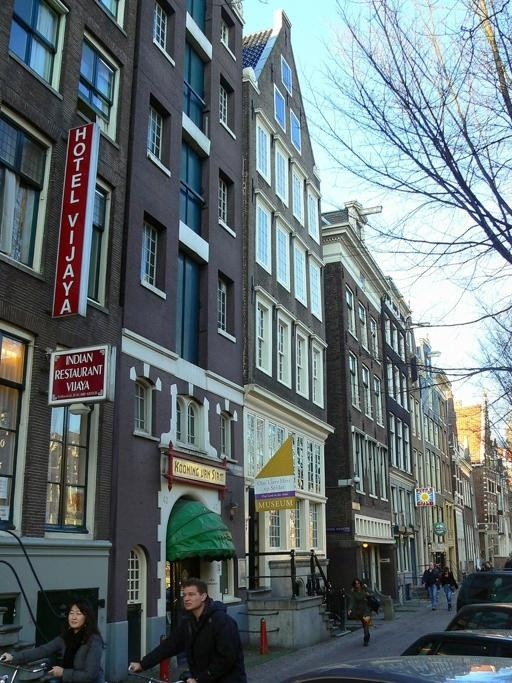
129,578 -> 247,683
423,557 -> 512,611
347,578 -> 375,646
0,600 -> 105,683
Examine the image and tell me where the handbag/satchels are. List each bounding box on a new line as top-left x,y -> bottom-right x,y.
364,585 -> 381,614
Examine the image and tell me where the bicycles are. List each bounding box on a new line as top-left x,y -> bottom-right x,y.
126,664 -> 185,682
0,657 -> 53,681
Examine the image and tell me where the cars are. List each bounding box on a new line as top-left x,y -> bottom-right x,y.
280,653 -> 512,682
401,627 -> 512,660
444,601 -> 512,633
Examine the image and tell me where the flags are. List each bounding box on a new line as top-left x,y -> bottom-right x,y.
254,436 -> 296,511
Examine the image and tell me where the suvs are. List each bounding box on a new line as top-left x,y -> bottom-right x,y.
456,566 -> 512,613
